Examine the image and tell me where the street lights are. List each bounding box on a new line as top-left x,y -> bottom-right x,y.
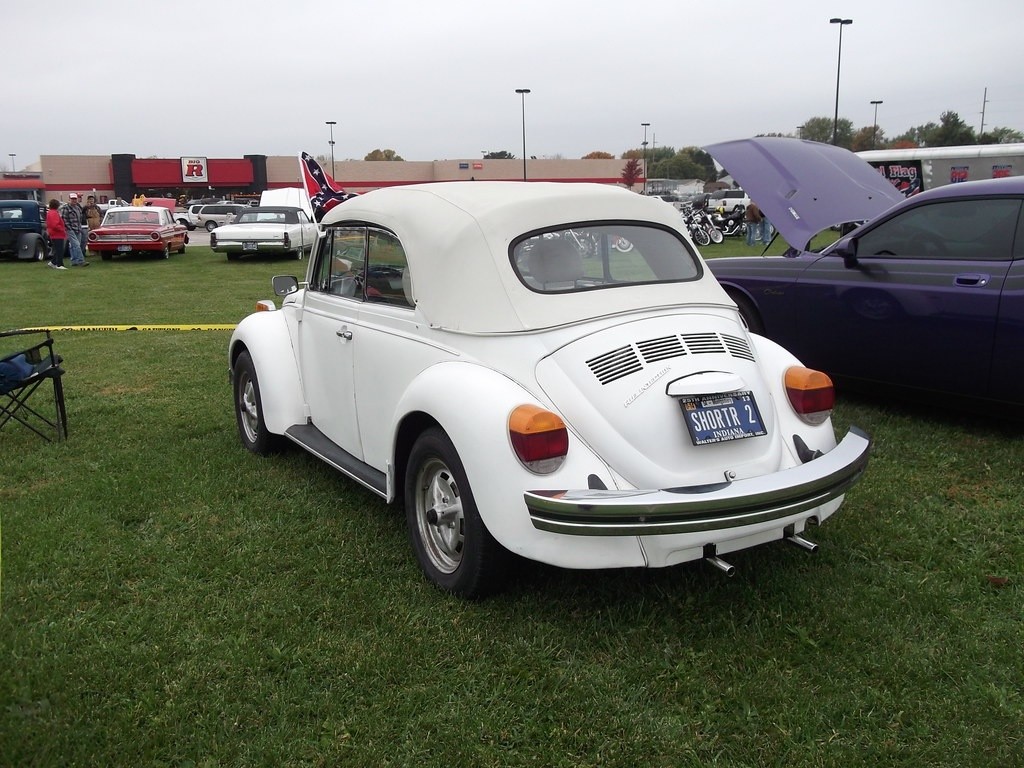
325,121 -> 338,181
869,100 -> 884,150
829,18 -> 853,146
640,122 -> 651,191
8,153 -> 17,172
796,126 -> 805,139
515,88 -> 531,182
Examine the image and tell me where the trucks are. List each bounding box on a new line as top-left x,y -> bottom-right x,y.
839,143 -> 1024,238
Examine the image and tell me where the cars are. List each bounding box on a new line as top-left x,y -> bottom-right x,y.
208,187 -> 336,263
0,199 -> 54,261
85,198 -> 190,260
226,178 -> 872,601
696,133 -> 1024,414
0,197 -> 260,232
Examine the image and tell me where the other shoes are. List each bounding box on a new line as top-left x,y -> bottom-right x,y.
81,261 -> 90,266
70,257 -> 75,260
55,264 -> 68,270
48,261 -> 56,268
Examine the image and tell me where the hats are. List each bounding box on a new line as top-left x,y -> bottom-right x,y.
76,192 -> 83,198
69,193 -> 77,199
87,194 -> 96,199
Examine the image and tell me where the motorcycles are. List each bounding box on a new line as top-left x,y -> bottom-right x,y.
643,186 -> 777,247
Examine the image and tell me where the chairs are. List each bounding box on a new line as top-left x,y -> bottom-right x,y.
0,328 -> 70,443
528,238 -> 586,283
118,213 -> 129,223
285,212 -> 298,224
961,214 -> 1011,257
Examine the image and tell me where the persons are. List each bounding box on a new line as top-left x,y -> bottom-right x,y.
745,197 -> 763,247
61,193 -> 90,268
758,208 -> 772,246
46,198 -> 69,269
82,194 -> 104,256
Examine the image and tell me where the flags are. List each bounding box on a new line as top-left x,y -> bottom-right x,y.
299,151 -> 361,225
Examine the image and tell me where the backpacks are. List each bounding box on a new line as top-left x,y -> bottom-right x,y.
758,208 -> 766,217
